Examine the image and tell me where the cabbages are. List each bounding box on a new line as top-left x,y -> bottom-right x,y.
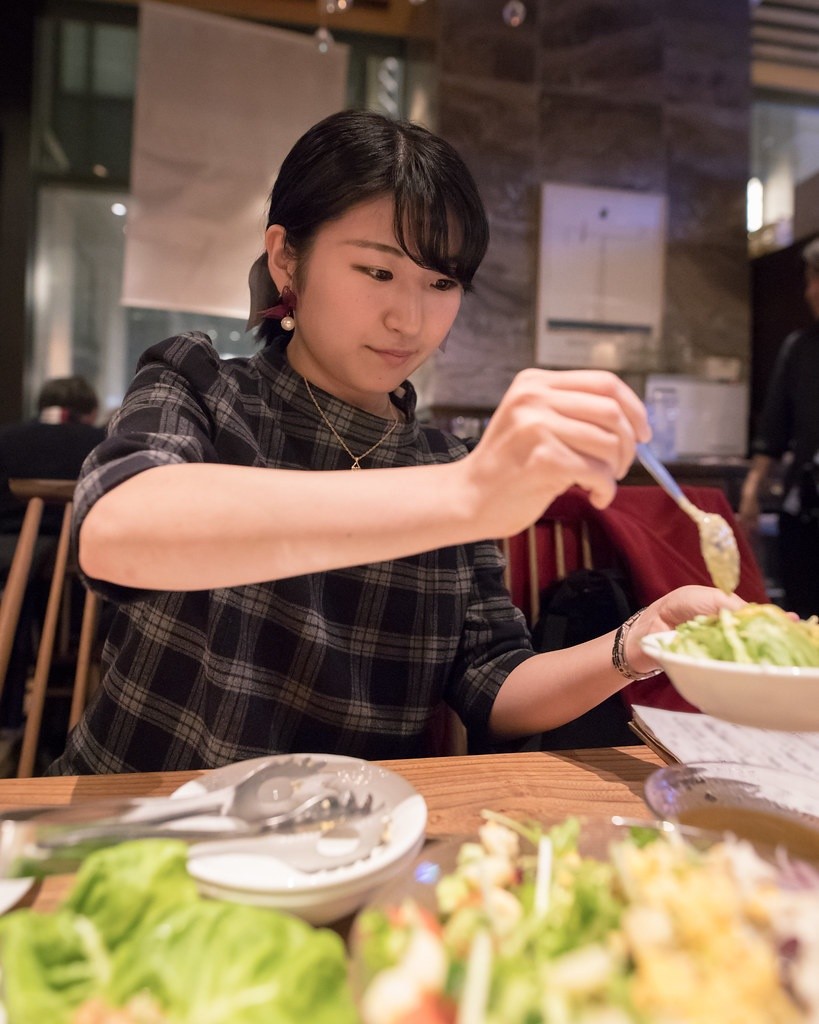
1,838 -> 357,1024
670,604 -> 818,668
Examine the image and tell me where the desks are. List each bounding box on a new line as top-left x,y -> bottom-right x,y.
0,744 -> 713,914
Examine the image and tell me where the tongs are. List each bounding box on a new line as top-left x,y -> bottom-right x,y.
32,757 -> 371,850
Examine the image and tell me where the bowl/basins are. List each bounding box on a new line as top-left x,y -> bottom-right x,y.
644,762 -> 819,871
349,816 -> 819,1024
638,630 -> 819,734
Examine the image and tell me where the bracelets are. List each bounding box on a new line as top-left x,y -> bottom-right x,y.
613,604 -> 664,682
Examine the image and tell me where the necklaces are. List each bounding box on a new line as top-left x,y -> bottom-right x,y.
302,374 -> 401,469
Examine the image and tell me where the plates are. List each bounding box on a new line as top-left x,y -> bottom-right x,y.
166,755 -> 428,926
0,822 -> 39,916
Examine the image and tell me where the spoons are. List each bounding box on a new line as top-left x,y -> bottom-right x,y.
637,445 -> 739,596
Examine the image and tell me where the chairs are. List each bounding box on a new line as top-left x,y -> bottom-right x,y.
0,477 -> 104,779
443,484 -> 769,758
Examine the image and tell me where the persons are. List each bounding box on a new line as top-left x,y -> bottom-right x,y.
737,239 -> 818,625
0,376 -> 128,673
50,106 -> 800,775
